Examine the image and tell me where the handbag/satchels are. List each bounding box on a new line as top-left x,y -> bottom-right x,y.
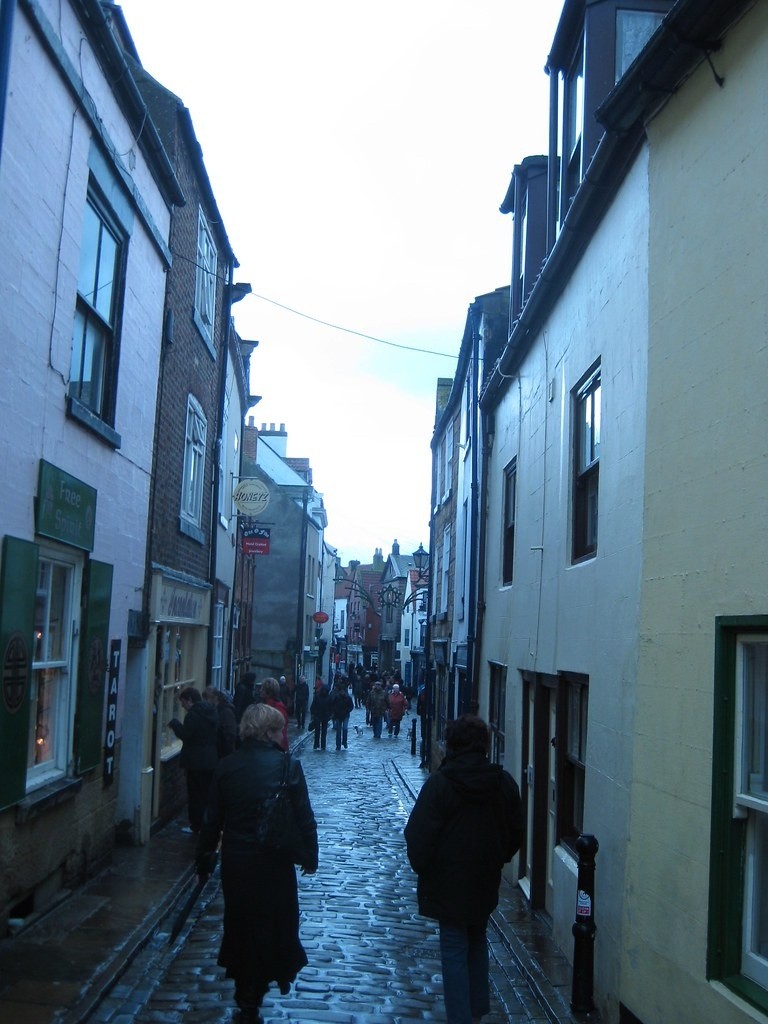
261,752 -> 303,875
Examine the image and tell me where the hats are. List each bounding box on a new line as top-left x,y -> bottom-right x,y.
393,684 -> 400,688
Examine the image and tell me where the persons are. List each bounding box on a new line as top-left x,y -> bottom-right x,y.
165,650 -> 426,834
404,713 -> 525,1024
199,703 -> 318,1024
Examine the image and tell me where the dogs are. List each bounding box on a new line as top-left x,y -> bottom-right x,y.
353,725 -> 363,737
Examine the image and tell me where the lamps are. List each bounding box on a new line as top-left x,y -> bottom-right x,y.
412,542 -> 431,581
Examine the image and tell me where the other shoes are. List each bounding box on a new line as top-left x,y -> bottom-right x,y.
336,746 -> 341,750
231,1008 -> 259,1024
181,827 -> 193,833
343,743 -> 347,748
278,969 -> 296,995
